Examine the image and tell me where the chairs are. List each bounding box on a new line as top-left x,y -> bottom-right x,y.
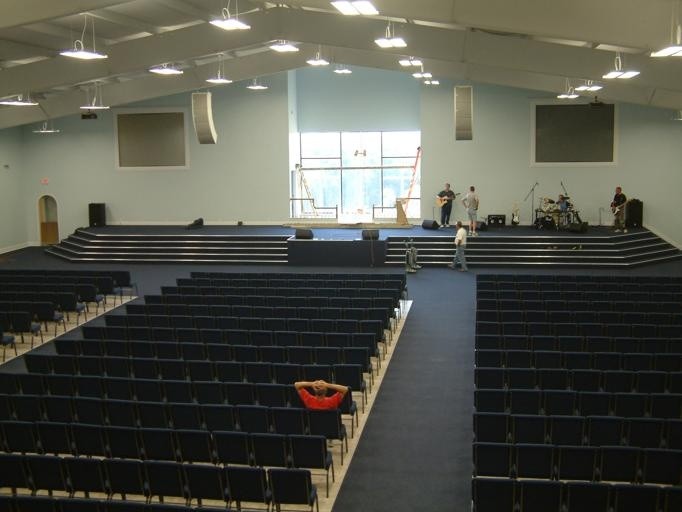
472,275 -> 681,509
0,269 -> 405,512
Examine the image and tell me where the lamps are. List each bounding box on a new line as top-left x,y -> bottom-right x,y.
374,20 -> 408,49
412,67 -> 432,79
204,53 -> 233,85
555,1 -> 681,103
329,2 -> 380,16
78,82 -> 112,112
397,55 -> 423,68
59,12 -> 109,62
245,76 -> 269,91
0,90 -> 39,108
30,119 -> 65,137
334,63 -> 353,76
147,63 -> 185,76
424,78 -> 440,87
306,48 -> 330,67
208,0 -> 252,33
269,39 -> 300,54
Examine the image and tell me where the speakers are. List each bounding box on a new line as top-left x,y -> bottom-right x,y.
296,229 -> 313,239
567,224 -> 586,233
625,204 -> 642,228
422,220 -> 440,229
472,222 -> 487,231
454,86 -> 474,141
362,230 -> 379,240
89,203 -> 105,227
192,92 -> 217,144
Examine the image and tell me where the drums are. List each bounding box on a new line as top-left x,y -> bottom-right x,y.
542,215 -> 555,228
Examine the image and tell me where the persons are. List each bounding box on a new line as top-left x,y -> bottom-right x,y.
294,379 -> 349,424
436,183 -> 456,228
538,196 -> 554,212
610,186 -> 628,233
447,221 -> 472,274
460,184 -> 480,238
556,195 -> 570,212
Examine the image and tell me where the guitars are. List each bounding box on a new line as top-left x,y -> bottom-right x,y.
612,200 -> 630,215
512,203 -> 520,224
436,192 -> 462,207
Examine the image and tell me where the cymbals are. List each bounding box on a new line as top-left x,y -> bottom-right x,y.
561,197 -> 570,200
548,199 -> 555,203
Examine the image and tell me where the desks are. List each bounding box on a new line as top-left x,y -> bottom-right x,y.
284,234 -> 389,269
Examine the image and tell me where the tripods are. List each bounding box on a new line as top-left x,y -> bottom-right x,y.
527,191 -> 537,229
